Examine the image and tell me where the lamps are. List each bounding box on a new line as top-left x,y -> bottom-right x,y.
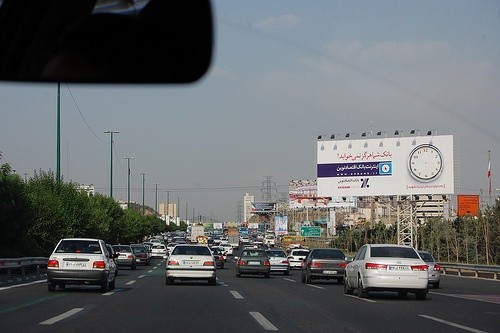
345,133 -> 357,137
313,196 -> 322,201
329,196 -> 333,201
342,196 -> 347,201
393,130 -> 404,138
374,194 -> 380,202
358,196 -> 364,202
362,131 -> 371,139
377,131 -> 387,138
317,132 -> 327,140
413,194 -> 420,201
330,133 -> 341,139
411,128 -> 420,137
442,193 -> 449,201
389,194 -> 394,201
402,195 -> 408,201
428,194 -> 434,200
426,128 -> 439,135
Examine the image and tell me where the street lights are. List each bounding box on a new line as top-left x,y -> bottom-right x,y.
104,130 -> 121,196
123,156 -> 132,208
140,172 -> 146,213
166,190 -> 172,204
153,182 -> 160,215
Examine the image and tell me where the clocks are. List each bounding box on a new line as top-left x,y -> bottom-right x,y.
407,144 -> 444,183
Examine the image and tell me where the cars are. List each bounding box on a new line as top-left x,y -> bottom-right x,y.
211,245 -> 228,261
219,243 -> 234,255
167,243 -> 179,252
265,250 -> 290,275
142,224 -> 304,252
110,245 -> 137,270
298,248 -> 349,283
287,248 -> 310,269
163,245 -> 217,286
343,243 -> 430,297
45,238 -> 117,290
418,251 -> 441,287
234,249 -> 271,278
151,244 -> 168,259
211,248 -> 225,269
130,244 -> 150,267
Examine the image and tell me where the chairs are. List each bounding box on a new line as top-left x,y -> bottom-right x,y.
178,250 -> 187,255
